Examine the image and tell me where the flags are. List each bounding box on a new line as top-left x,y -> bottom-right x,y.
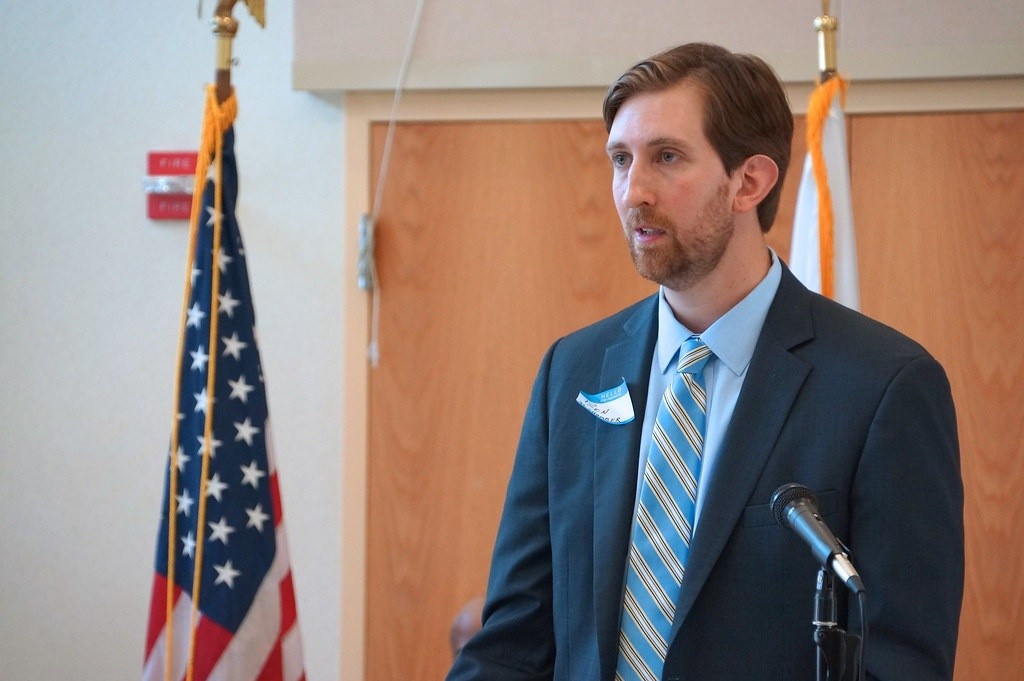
137,87 -> 307,681
788,75 -> 860,311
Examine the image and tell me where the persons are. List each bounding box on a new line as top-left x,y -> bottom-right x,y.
437,42 -> 966,681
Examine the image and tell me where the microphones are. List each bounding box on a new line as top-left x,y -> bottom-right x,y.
770,483 -> 866,593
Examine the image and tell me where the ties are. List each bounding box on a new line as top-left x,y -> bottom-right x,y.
612,337 -> 714,681
